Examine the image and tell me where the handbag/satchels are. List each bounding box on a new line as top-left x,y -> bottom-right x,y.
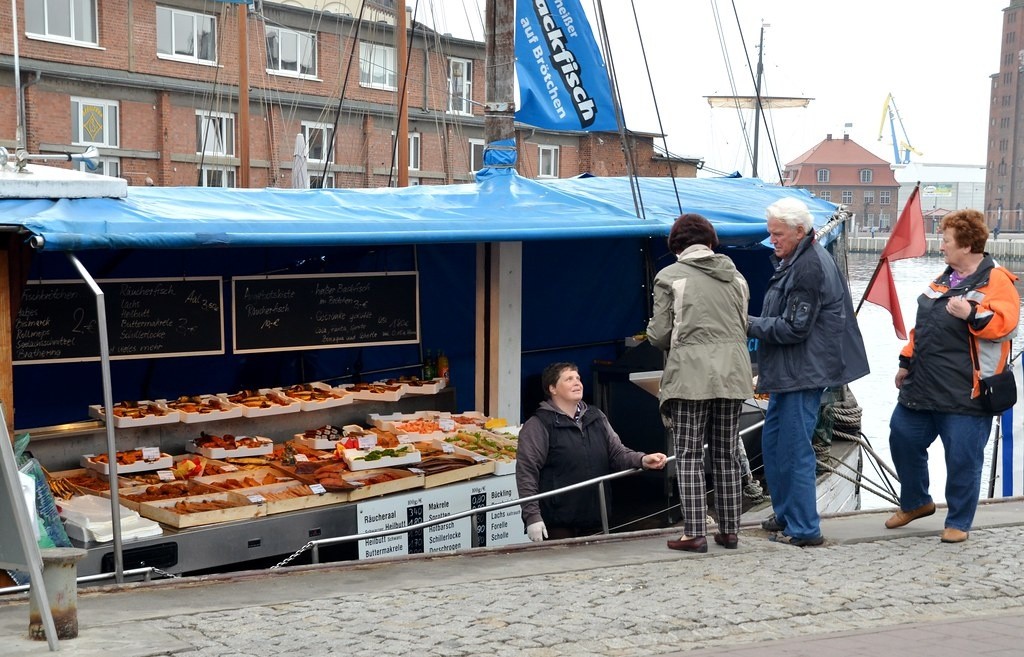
979,365 -> 1018,412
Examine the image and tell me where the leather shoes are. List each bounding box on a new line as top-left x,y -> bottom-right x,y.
668,534 -> 708,552
714,533 -> 738,548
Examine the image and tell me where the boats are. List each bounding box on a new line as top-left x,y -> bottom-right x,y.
2,0 -> 869,591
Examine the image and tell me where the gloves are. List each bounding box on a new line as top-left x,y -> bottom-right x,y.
526,521 -> 548,541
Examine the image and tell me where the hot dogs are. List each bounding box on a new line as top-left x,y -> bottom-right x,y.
443,430 -> 518,461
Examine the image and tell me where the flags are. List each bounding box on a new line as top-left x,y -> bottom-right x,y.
865,185 -> 927,341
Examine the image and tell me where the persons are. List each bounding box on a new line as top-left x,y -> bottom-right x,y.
879,224 -> 890,233
746,195 -> 870,548
870,226 -> 877,238
645,214 -> 755,554
884,209 -> 1021,545
514,360 -> 670,543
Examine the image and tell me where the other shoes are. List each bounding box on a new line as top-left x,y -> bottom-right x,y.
885,499 -> 935,529
761,517 -> 785,529
942,526 -> 968,542
768,531 -> 824,546
